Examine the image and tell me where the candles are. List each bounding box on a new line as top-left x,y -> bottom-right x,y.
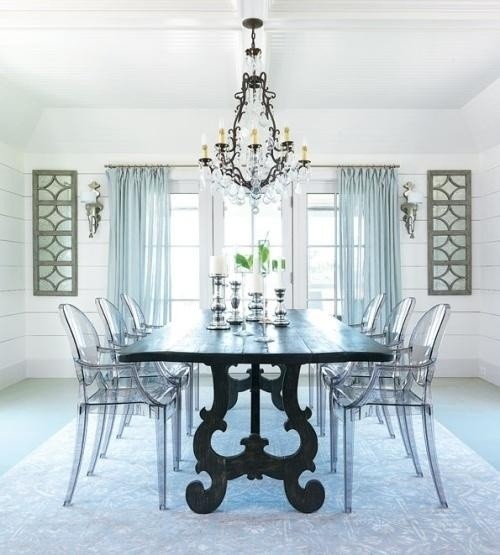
246,273 -> 264,293
271,271 -> 288,288
209,255 -> 227,275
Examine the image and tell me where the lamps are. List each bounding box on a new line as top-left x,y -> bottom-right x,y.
400,181 -> 418,239
84,181 -> 103,238
196,17 -> 314,215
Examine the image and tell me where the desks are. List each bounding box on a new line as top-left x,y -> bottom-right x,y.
118,307 -> 393,513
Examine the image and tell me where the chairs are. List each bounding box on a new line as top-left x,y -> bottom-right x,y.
321,297 -> 416,457
58,303 -> 179,510
331,304 -> 452,514
96,297 -> 191,461
120,293 -> 200,428
309,292 -> 385,425
